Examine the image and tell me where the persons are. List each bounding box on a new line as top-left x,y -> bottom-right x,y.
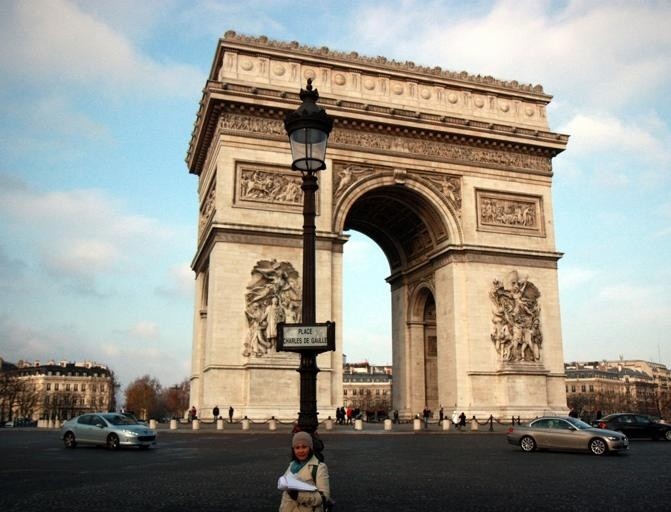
213,404 -> 220,424
456,411 -> 467,431
481,198 -> 537,228
191,405 -> 197,420
229,405 -> 234,424
423,405 -> 432,428
438,407 -> 443,425
336,404 -> 387,427
120,405 -> 127,413
276,431 -> 341,511
332,164 -> 373,198
489,273 -> 541,363
393,407 -> 401,424
243,262 -> 301,357
239,168 -> 303,204
424,173 -> 456,203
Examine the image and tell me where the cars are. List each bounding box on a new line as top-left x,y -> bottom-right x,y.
506,415 -> 632,457
58,410 -> 158,452
4,416 -> 37,428
592,412 -> 670,443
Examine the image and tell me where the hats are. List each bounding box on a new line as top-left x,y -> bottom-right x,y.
292,431 -> 314,451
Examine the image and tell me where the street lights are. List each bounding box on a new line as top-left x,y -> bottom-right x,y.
277,77 -> 334,464
